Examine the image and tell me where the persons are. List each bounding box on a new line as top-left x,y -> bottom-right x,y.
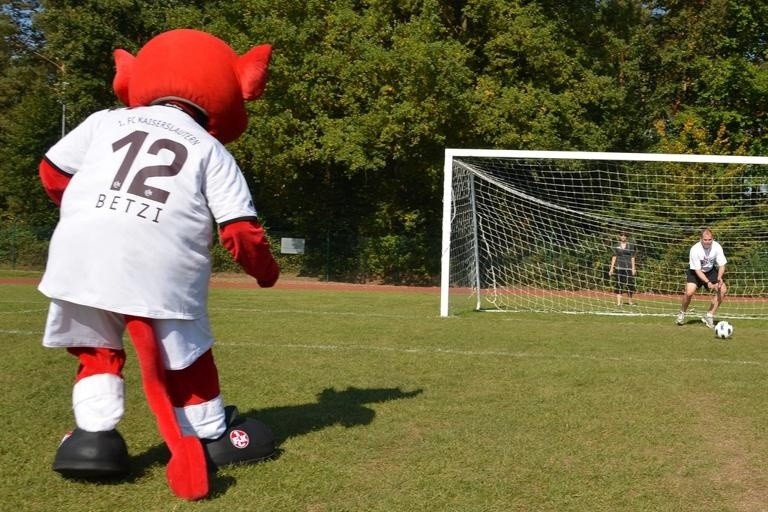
673,228 -> 728,330
607,229 -> 641,306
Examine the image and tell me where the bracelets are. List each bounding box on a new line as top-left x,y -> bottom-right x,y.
705,280 -> 710,286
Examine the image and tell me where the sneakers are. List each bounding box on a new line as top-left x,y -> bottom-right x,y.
701,313 -> 715,329
674,310 -> 685,326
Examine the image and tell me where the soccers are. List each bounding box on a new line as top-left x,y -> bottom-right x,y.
715,321 -> 734,338
